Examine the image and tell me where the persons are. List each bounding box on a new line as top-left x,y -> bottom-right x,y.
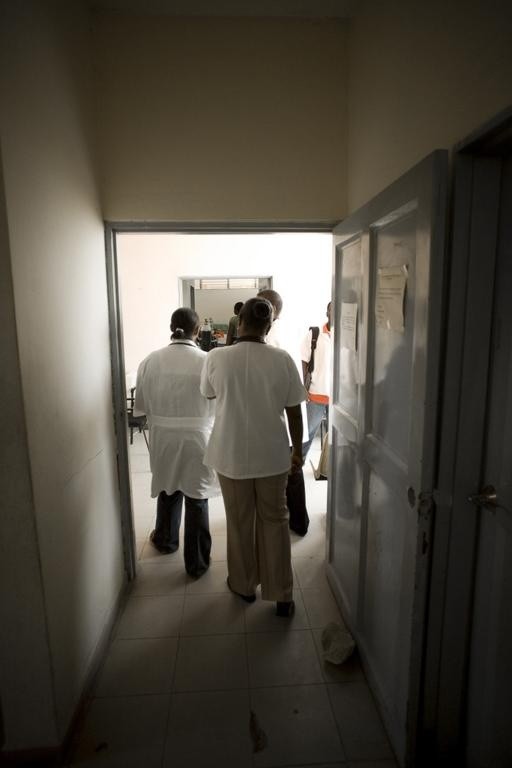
256,290 -> 311,538
226,301 -> 244,345
198,295 -> 306,620
300,301 -> 332,481
129,307 -> 215,580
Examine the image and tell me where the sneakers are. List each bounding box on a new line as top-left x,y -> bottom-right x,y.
291,525 -> 308,537
149,529 -> 211,582
227,575 -> 296,619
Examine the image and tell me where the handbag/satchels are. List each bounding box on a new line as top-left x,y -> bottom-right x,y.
304,371 -> 312,393
